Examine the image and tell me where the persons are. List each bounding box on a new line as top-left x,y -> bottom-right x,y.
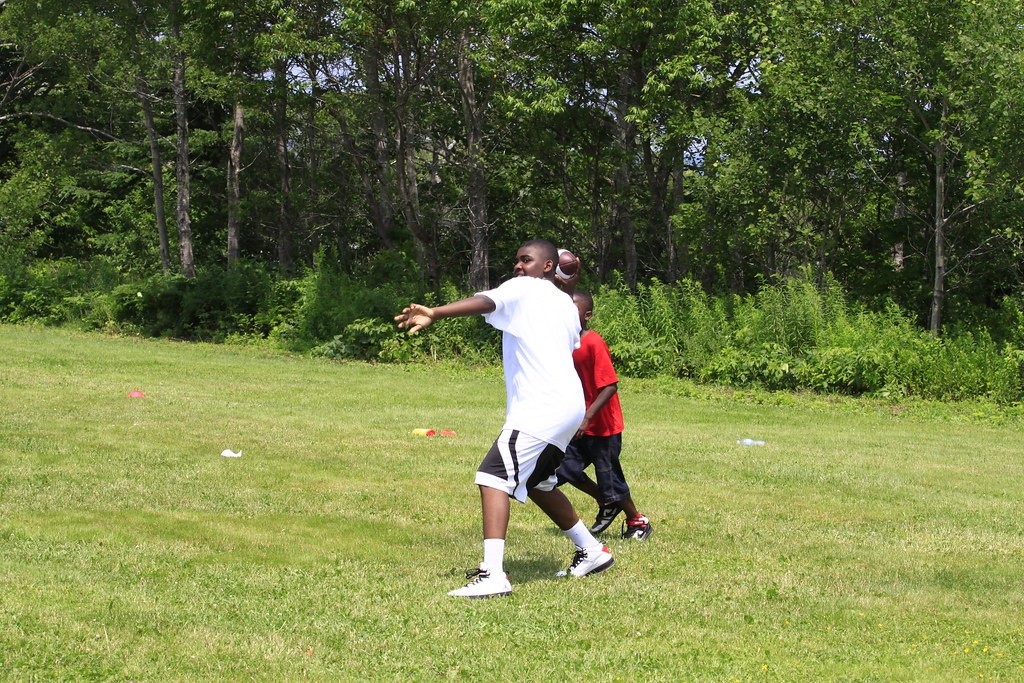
394,238 -> 616,600
554,289 -> 653,542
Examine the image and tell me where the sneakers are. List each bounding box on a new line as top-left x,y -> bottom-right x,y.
591,501 -> 623,533
446,563 -> 513,598
556,544 -> 616,578
621,514 -> 652,541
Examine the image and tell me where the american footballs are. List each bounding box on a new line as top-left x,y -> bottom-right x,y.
555,248 -> 578,280
